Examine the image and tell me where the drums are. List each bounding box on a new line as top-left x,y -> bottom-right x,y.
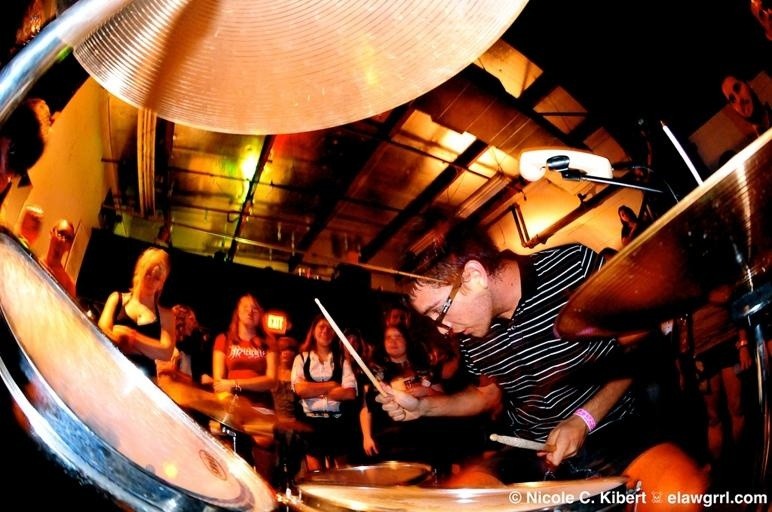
161,384 -> 312,431
0,223 -> 285,512
285,473 -> 641,512
301,460 -> 437,487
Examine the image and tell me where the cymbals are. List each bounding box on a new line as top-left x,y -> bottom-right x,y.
54,0 -> 531,135
554,127 -> 772,341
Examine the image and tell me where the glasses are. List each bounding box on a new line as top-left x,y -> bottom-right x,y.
423,272 -> 461,331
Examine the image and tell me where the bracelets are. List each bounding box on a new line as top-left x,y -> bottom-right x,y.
573,408 -> 597,432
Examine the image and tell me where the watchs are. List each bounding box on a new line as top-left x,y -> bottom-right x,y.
737,341 -> 748,350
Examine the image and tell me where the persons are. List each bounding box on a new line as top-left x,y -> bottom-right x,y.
376,210 -> 710,512
750,0 -> 772,41
98,246 -> 176,386
153,209 -> 173,247
153,293 -> 500,480
36,220 -> 77,304
3,1 -> 70,189
618,205 -> 640,246
12,206 -> 45,250
719,72 -> 772,136
692,286 -> 752,448
